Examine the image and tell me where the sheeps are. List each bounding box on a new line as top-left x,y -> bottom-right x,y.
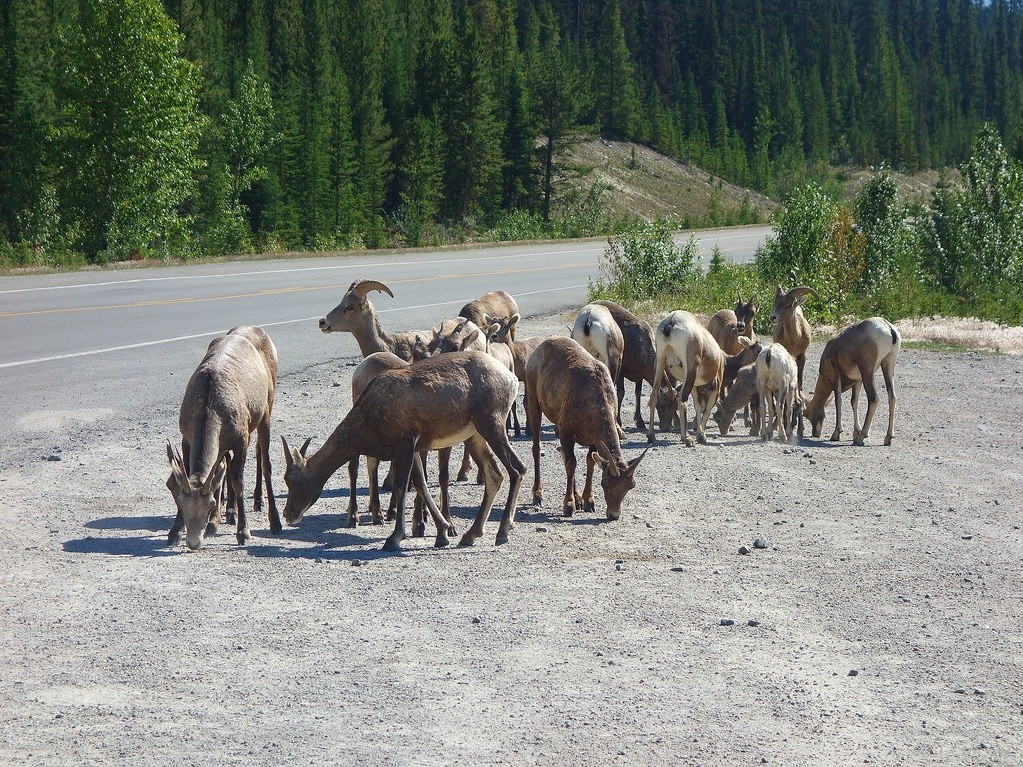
164,277 -> 902,549
